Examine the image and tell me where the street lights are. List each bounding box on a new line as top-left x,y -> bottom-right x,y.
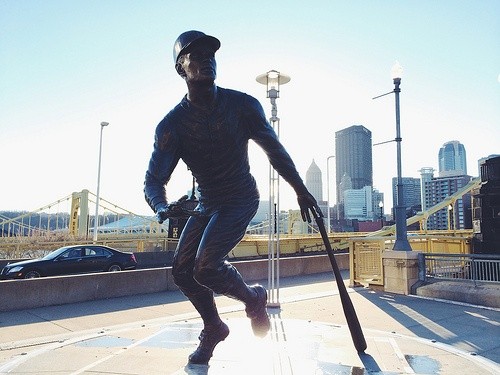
255,69 -> 291,309
326,155 -> 337,233
93,121 -> 110,245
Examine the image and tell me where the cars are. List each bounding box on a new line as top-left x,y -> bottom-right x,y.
0,244 -> 137,280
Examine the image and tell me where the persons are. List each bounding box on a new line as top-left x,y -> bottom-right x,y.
143,30 -> 325,364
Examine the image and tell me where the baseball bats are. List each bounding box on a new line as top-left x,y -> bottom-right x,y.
315,217 -> 368,353
